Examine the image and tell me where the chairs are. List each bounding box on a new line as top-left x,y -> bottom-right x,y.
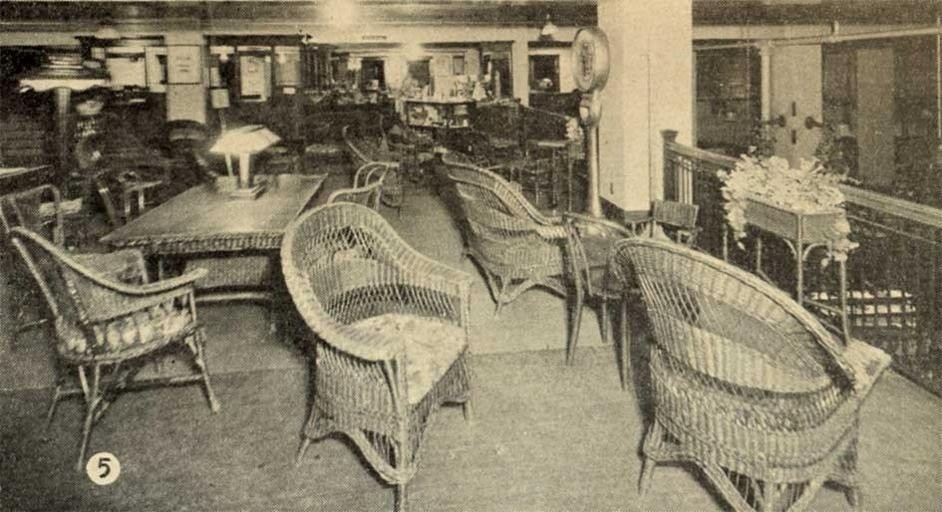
562,212 -> 637,378
442,154 -> 576,314
609,238 -> 894,512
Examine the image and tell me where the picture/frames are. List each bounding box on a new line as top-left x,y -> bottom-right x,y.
167,44 -> 204,85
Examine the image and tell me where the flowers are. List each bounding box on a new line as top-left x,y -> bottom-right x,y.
719,145 -> 861,268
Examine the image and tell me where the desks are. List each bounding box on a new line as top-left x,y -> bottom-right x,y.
722,197 -> 852,347
